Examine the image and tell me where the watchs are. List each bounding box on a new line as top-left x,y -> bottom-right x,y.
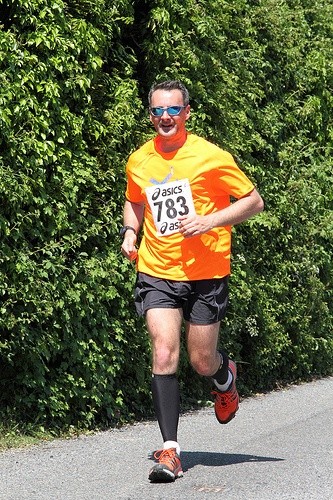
119,226 -> 138,239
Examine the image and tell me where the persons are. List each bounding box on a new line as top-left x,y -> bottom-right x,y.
118,80 -> 266,483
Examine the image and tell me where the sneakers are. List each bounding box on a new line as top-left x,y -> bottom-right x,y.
211,359 -> 239,424
148,448 -> 183,483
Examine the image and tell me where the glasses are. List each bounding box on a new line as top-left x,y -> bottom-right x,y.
148,105 -> 186,117
